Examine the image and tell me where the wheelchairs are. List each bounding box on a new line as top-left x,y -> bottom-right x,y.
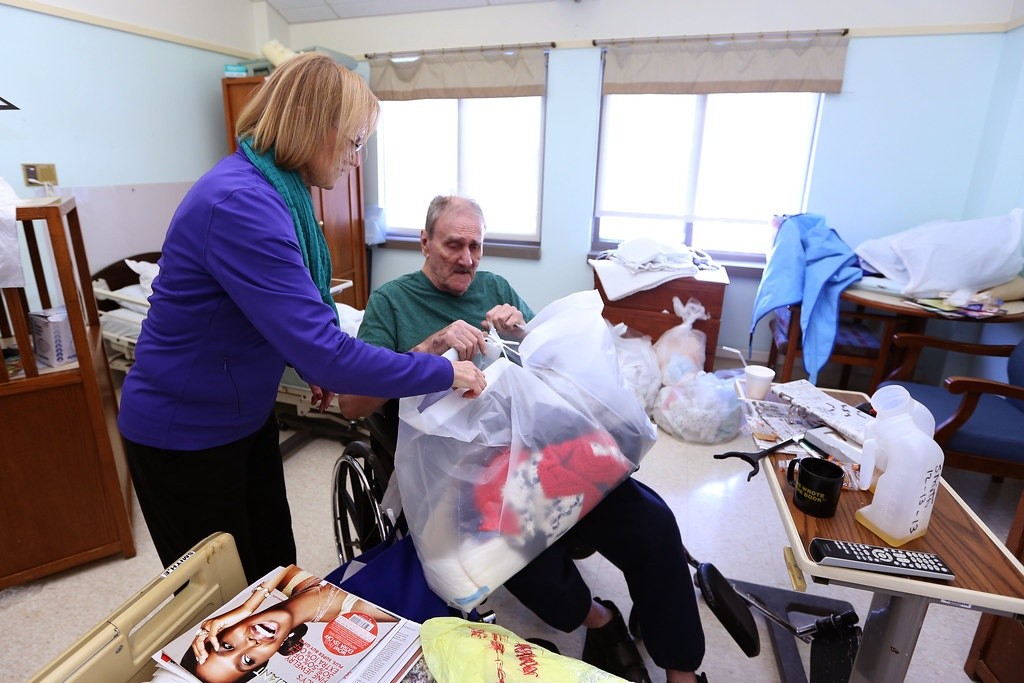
330,412 -> 761,659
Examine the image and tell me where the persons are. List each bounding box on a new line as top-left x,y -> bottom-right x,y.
336,196 -> 727,683
115,55 -> 487,596
180,563 -> 400,683
973,270 -> 1024,404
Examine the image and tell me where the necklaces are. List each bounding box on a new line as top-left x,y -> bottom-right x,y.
290,576 -> 342,625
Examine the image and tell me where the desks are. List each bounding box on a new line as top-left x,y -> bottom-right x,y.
737,380 -> 1024,683
836,289 -> 1024,387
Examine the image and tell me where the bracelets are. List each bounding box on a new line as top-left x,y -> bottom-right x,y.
251,585 -> 271,599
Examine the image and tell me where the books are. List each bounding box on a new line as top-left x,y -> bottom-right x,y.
803,426 -> 864,467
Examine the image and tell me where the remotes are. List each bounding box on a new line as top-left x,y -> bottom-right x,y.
808,537 -> 955,581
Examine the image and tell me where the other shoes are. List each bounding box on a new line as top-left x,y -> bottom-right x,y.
588,596 -> 650,683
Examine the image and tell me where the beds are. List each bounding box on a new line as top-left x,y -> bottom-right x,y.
92,251 -> 370,438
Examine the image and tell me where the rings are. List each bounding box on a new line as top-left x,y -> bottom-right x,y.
195,627 -> 210,638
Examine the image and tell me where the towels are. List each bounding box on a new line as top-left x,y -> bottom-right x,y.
588,236 -> 721,302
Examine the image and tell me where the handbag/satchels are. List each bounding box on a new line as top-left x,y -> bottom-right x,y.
394,288 -> 659,615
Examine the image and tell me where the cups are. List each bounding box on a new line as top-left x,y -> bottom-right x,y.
786,456 -> 846,519
744,364 -> 776,401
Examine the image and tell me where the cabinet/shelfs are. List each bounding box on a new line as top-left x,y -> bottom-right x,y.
592,263 -> 730,372
221,76 -> 367,310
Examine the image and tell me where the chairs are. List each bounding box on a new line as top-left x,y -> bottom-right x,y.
877,333 -> 1024,485
769,215 -> 906,393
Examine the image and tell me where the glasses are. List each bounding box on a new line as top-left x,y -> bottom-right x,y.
328,122 -> 364,152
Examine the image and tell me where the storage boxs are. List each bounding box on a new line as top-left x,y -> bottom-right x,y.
29,304 -> 79,367
